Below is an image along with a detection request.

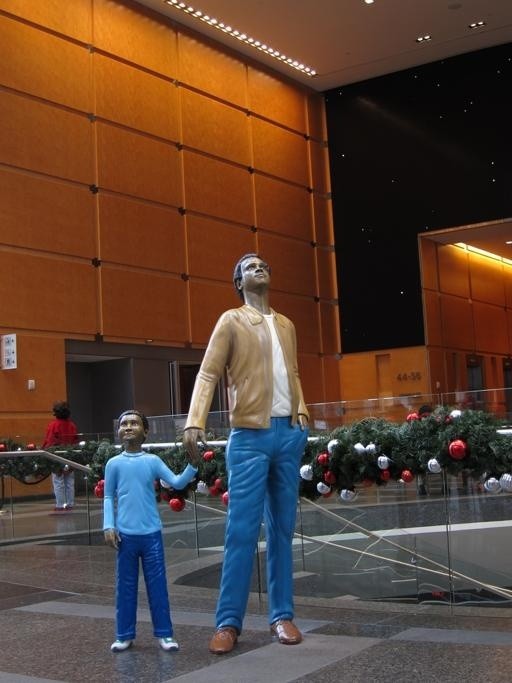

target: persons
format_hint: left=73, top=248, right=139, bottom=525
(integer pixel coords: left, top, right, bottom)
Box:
left=184, top=253, right=310, bottom=653
left=103, top=409, right=200, bottom=652
left=42, top=400, right=78, bottom=509
